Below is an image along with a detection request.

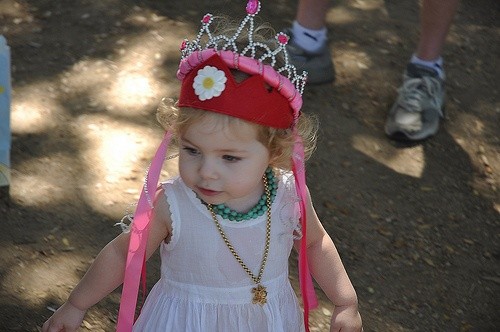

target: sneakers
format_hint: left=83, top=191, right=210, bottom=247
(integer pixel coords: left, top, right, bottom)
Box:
left=273, top=28, right=337, bottom=92
left=384, top=72, right=448, bottom=141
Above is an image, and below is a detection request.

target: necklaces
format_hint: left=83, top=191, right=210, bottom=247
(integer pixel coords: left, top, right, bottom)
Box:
left=192, top=167, right=278, bottom=304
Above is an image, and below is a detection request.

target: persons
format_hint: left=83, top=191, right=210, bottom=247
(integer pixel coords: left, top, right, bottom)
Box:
left=238, top=0, right=459, bottom=142
left=41, top=16, right=362, bottom=332
